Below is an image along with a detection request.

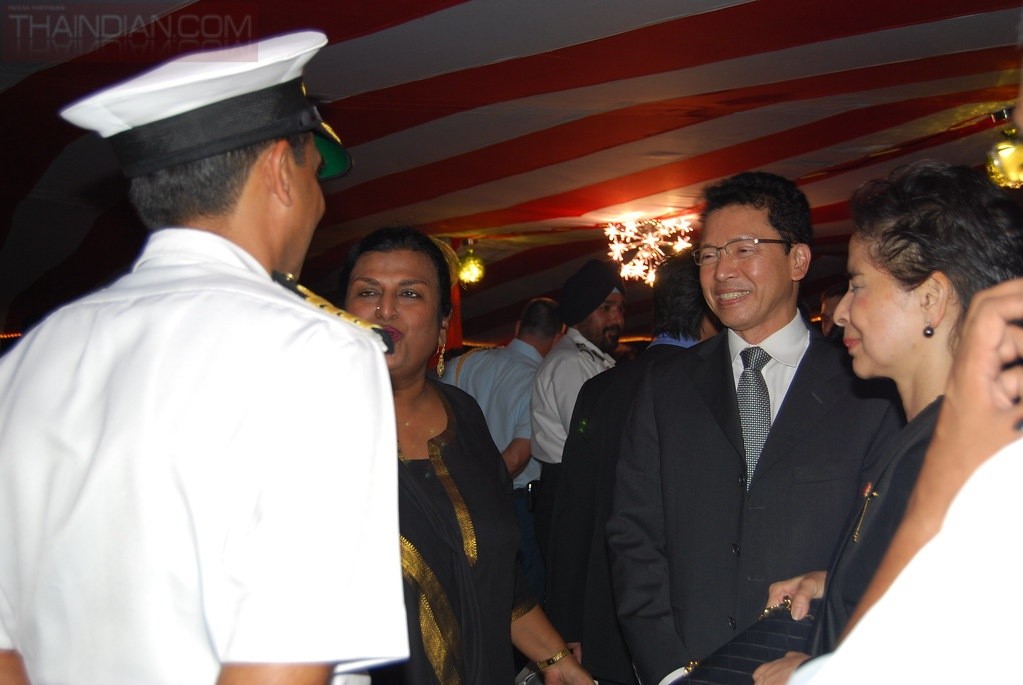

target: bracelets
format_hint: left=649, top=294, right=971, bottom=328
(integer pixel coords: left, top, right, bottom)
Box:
left=537, top=648, right=570, bottom=670
left=659, top=661, right=699, bottom=685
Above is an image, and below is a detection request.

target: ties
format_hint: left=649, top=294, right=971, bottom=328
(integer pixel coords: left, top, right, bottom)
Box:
left=736, top=346, right=773, bottom=493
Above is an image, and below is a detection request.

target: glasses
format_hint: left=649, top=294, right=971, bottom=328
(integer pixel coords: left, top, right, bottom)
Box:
left=693, top=237, right=792, bottom=267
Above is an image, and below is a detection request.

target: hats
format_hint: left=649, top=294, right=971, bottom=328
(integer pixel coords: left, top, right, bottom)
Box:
left=49, top=30, right=357, bottom=185
left=558, top=258, right=617, bottom=326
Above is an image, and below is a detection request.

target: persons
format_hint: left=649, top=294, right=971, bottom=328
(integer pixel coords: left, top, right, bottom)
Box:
left=536, top=251, right=722, bottom=685
left=603, top=172, right=903, bottom=685
left=821, top=286, right=847, bottom=337
left=440, top=296, right=564, bottom=600
left=1, top=32, right=410, bottom=684
left=807, top=157, right=1023, bottom=658
left=336, top=224, right=602, bottom=685
left=525, top=258, right=626, bottom=500
left=787, top=276, right=1023, bottom=685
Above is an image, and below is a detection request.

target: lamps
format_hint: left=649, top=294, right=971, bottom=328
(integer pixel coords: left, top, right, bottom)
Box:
left=602, top=220, right=694, bottom=287
left=987, top=108, right=1023, bottom=189
left=456, top=239, right=484, bottom=285
